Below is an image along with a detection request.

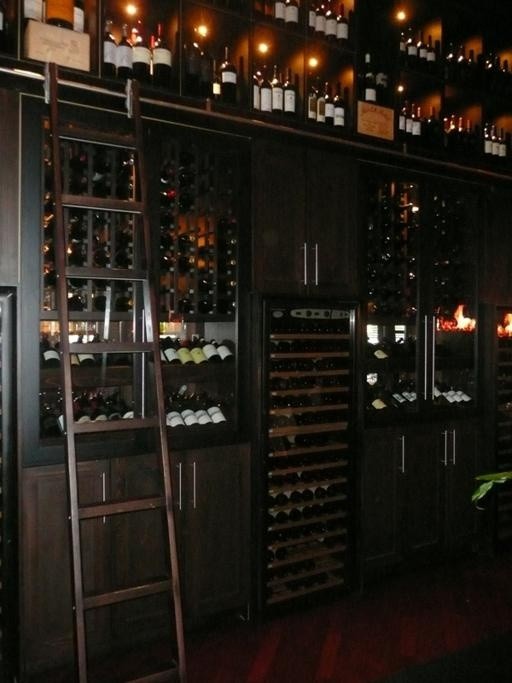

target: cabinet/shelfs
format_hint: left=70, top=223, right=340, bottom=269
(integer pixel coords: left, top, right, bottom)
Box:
left=0, top=0, right=511, bottom=682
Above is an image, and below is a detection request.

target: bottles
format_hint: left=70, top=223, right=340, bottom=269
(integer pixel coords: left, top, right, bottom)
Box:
left=23, top=1, right=88, bottom=33
left=365, top=180, right=473, bottom=412
left=401, top=28, right=437, bottom=66
left=103, top=18, right=174, bottom=90
left=262, top=318, right=353, bottom=593
left=357, top=51, right=392, bottom=105
left=183, top=37, right=238, bottom=103
left=307, top=71, right=347, bottom=126
left=256, top=1, right=349, bottom=42
left=444, top=42, right=509, bottom=159
left=400, top=99, right=438, bottom=146
left=41, top=128, right=238, bottom=313
left=253, top=64, right=298, bottom=114
left=38, top=336, right=234, bottom=434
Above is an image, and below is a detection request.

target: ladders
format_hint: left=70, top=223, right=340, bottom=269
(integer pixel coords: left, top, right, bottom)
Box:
left=46, top=61, right=188, bottom=682
